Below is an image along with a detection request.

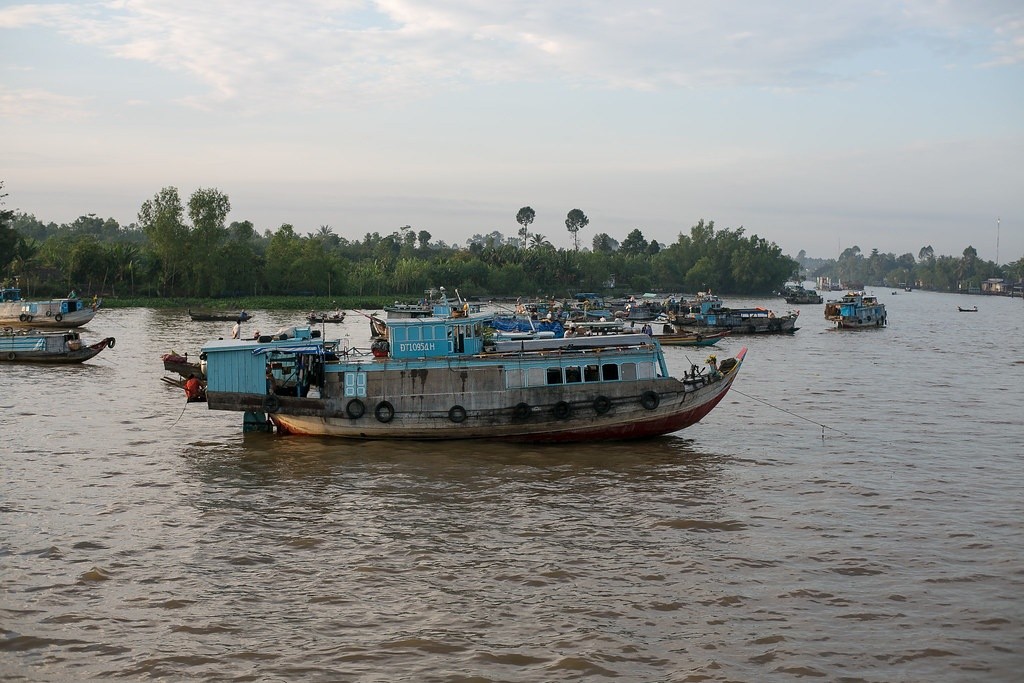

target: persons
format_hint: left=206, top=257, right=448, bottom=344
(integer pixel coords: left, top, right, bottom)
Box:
left=309, top=310, right=317, bottom=319
left=185, top=374, right=204, bottom=399
left=335, top=308, right=340, bottom=317
left=240, top=308, right=247, bottom=316
left=564, top=314, right=652, bottom=337
left=231, top=320, right=241, bottom=339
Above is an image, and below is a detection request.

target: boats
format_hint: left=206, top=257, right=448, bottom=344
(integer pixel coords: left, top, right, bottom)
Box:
left=0, top=275, right=102, bottom=329
left=158, top=311, right=749, bottom=445
left=354, top=282, right=802, bottom=348
left=0, top=324, right=117, bottom=365
left=187, top=308, right=254, bottom=321
left=822, top=289, right=888, bottom=328
left=304, top=311, right=346, bottom=324
left=771, top=281, right=825, bottom=305
left=956, top=305, right=979, bottom=313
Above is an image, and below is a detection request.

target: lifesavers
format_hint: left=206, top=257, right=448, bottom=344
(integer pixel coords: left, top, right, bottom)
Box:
left=108, top=337, right=115, bottom=348
left=594, top=396, right=611, bottom=413
left=55, top=314, right=62, bottom=322
left=449, top=405, right=467, bottom=423
left=20, top=314, right=32, bottom=323
left=641, top=390, right=660, bottom=410
left=263, top=395, right=280, bottom=413
left=727, top=324, right=733, bottom=330
left=858, top=319, right=862, bottom=323
left=697, top=336, right=702, bottom=342
left=750, top=326, right=756, bottom=331
left=46, top=311, right=51, bottom=317
left=375, top=400, right=395, bottom=423
left=867, top=316, right=871, bottom=321
left=7, top=352, right=16, bottom=360
left=554, top=400, right=570, bottom=419
left=346, top=399, right=365, bottom=418
left=515, top=402, right=530, bottom=418
